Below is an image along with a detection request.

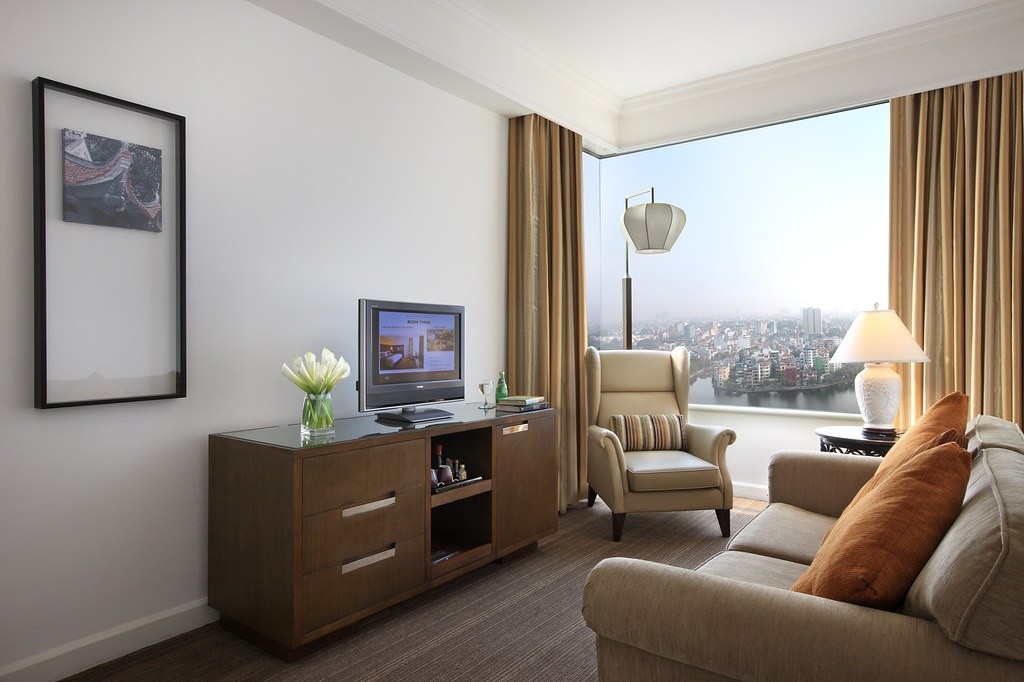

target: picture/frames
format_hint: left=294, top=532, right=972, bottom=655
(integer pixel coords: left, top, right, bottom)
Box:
left=31, top=76, right=188, bottom=408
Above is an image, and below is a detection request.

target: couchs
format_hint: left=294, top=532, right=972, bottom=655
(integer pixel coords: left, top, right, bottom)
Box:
left=582, top=415, right=1024, bottom=682
left=584, top=346, right=735, bottom=542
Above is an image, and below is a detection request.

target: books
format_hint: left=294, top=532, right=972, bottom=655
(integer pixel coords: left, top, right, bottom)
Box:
left=496, top=396, right=549, bottom=412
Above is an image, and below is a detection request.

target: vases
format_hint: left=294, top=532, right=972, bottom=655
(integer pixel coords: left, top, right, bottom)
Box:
left=302, top=393, right=337, bottom=438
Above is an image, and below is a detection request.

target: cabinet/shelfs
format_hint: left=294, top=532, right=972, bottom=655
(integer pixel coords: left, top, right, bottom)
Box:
left=206, top=402, right=561, bottom=661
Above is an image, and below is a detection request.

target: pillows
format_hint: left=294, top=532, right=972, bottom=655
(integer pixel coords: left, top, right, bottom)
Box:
left=610, top=416, right=690, bottom=452
left=792, top=444, right=971, bottom=607
left=873, top=391, right=973, bottom=472
left=822, top=431, right=969, bottom=538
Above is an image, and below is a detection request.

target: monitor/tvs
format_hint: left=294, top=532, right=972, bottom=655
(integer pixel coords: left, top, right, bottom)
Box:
left=357, top=299, right=466, bottom=423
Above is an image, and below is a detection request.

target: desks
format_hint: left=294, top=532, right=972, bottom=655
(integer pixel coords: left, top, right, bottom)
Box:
left=814, top=426, right=900, bottom=457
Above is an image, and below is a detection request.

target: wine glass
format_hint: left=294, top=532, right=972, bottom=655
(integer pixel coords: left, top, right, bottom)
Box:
left=478, top=380, right=494, bottom=409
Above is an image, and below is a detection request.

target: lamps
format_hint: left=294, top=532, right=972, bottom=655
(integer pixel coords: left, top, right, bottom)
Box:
left=827, top=303, right=933, bottom=436
left=622, top=188, right=686, bottom=349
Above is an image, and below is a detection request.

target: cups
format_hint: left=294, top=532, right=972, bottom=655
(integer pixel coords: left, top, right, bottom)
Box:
left=437, top=465, right=453, bottom=484
left=431, top=469, right=438, bottom=486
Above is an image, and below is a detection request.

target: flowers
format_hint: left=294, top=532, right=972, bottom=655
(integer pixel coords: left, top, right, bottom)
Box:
left=279, top=349, right=350, bottom=427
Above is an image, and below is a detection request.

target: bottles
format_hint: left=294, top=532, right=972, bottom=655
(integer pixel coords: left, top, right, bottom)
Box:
left=435, top=444, right=467, bottom=490
left=495, top=371, right=509, bottom=405
